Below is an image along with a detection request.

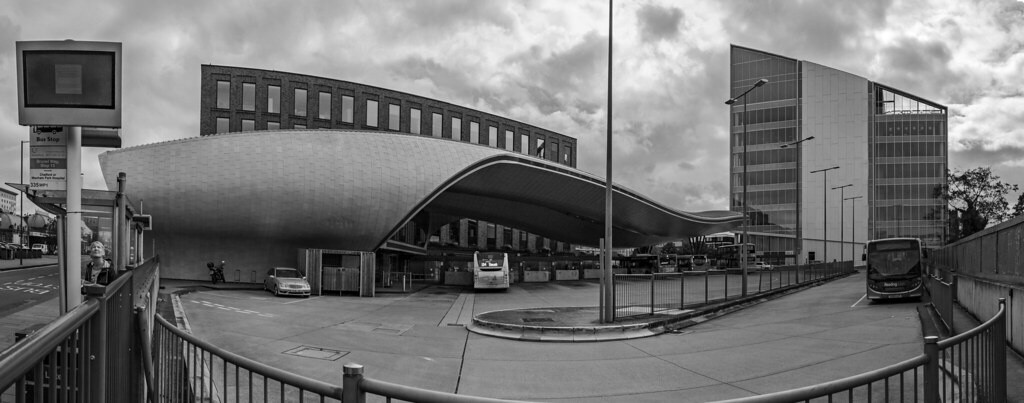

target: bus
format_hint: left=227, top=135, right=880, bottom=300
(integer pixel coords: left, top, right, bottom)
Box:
left=715, top=243, right=757, bottom=269
left=627, top=253, right=678, bottom=280
left=677, top=254, right=708, bottom=273
left=862, top=237, right=928, bottom=302
left=473, top=251, right=510, bottom=292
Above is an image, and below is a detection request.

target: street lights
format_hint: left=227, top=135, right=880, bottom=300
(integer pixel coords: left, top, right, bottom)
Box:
left=831, top=183, right=854, bottom=277
left=779, top=134, right=815, bottom=285
left=843, top=195, right=864, bottom=273
left=810, top=166, right=841, bottom=278
left=724, top=77, right=770, bottom=298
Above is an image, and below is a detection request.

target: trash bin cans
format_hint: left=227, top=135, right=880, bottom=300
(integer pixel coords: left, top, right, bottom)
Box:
left=15, top=322, right=81, bottom=403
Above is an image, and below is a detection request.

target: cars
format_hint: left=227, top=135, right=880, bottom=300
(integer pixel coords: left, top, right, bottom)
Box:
left=264, top=267, right=311, bottom=298
left=0, top=241, right=49, bottom=256
left=756, top=262, right=774, bottom=269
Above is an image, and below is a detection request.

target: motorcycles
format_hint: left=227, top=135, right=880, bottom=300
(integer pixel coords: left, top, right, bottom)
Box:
left=207, top=260, right=227, bottom=284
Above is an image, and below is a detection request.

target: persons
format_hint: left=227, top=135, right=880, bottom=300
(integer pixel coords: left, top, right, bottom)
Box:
left=86, top=240, right=116, bottom=288
